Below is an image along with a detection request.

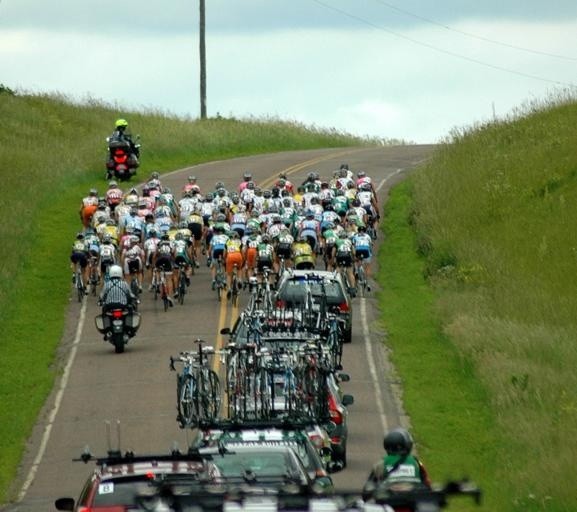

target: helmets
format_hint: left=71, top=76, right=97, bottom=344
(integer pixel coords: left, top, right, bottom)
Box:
left=109, top=264, right=123, bottom=279
left=76, top=171, right=202, bottom=244
left=384, top=428, right=412, bottom=454
left=206, top=164, right=372, bottom=243
left=115, top=119, right=128, bottom=129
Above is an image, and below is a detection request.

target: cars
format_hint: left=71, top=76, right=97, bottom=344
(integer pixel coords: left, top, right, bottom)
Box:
left=55, top=346, right=354, bottom=512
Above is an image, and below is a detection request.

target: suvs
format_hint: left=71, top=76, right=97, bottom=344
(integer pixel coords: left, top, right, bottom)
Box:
left=273, top=265, right=357, bottom=344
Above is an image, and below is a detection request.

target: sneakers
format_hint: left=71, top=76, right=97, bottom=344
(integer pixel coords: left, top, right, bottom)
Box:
left=167, top=296, right=174, bottom=307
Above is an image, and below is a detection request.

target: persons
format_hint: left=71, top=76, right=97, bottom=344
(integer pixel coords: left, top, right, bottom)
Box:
left=69, top=163, right=377, bottom=347
left=105, top=118, right=138, bottom=180
left=362, top=428, right=427, bottom=492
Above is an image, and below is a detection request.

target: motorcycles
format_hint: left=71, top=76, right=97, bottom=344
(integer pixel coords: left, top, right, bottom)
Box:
left=90, top=279, right=142, bottom=354
left=363, top=476, right=433, bottom=511
left=105, top=133, right=142, bottom=181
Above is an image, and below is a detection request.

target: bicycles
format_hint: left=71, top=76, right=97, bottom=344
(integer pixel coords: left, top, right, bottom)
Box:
left=70, top=206, right=372, bottom=312
left=169, top=276, right=344, bottom=430
